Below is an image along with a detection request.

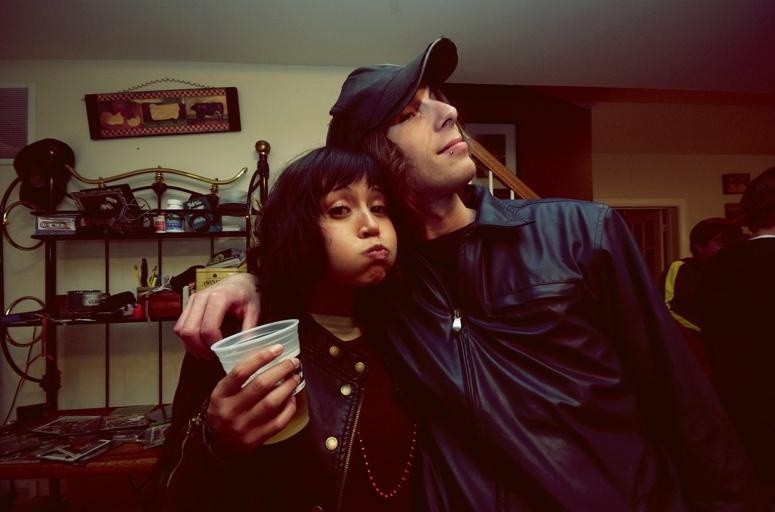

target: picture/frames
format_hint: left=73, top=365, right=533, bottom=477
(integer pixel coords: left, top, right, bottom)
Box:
left=461, top=122, right=517, bottom=191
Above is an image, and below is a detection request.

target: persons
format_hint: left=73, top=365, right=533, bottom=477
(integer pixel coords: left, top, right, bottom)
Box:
left=142, top=143, right=467, bottom=511
left=699, top=160, right=774, bottom=486
left=658, top=213, right=744, bottom=334
left=173, top=32, right=775, bottom=511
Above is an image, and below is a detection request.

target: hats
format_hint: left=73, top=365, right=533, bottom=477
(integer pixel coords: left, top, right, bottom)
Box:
left=326, top=36, right=460, bottom=150
left=13, top=138, right=77, bottom=211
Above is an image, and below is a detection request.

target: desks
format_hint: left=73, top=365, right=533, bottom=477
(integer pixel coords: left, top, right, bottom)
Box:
left=0, top=415, right=172, bottom=512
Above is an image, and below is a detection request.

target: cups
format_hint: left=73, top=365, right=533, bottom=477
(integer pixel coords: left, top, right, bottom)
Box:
left=210, top=317, right=310, bottom=445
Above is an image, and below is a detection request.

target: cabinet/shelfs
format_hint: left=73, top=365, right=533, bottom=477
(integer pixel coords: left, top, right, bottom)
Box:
left=0, top=137, right=272, bottom=413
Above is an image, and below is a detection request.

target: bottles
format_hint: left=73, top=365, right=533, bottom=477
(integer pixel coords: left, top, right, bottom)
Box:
left=156, top=199, right=185, bottom=233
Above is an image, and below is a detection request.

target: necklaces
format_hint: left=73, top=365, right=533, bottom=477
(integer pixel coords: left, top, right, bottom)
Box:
left=353, top=419, right=420, bottom=501
